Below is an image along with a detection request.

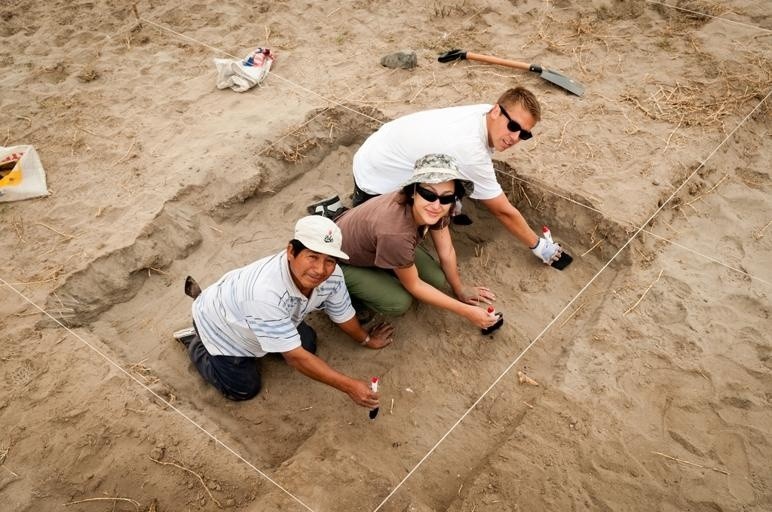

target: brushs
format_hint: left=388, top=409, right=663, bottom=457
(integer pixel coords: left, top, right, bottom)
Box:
left=481, top=307, right=504, bottom=335
left=185, top=276, right=202, bottom=300
left=543, top=226, right=573, bottom=271
left=369, top=377, right=379, bottom=419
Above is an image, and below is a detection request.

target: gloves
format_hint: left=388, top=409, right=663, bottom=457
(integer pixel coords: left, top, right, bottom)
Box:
left=450, top=196, right=463, bottom=217
left=529, top=238, right=559, bottom=264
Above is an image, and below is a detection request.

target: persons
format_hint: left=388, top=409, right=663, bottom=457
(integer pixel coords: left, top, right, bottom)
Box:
left=306, top=85, right=563, bottom=266
left=329, top=153, right=501, bottom=331
left=172, top=213, right=394, bottom=411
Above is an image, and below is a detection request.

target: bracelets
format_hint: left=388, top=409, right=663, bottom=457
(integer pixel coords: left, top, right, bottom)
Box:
left=359, top=333, right=371, bottom=347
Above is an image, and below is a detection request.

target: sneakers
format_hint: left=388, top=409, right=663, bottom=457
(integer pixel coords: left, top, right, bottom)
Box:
left=174, top=327, right=196, bottom=350
left=307, top=194, right=343, bottom=217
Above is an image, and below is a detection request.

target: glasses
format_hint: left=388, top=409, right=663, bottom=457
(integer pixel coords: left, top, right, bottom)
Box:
left=499, top=104, right=533, bottom=141
left=416, top=185, right=456, bottom=205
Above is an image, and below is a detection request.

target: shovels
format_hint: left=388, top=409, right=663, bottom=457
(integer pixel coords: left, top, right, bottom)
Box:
left=437, top=50, right=587, bottom=97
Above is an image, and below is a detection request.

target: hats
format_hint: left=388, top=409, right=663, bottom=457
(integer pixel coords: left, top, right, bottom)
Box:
left=293, top=214, right=351, bottom=260
left=401, top=152, right=475, bottom=202
